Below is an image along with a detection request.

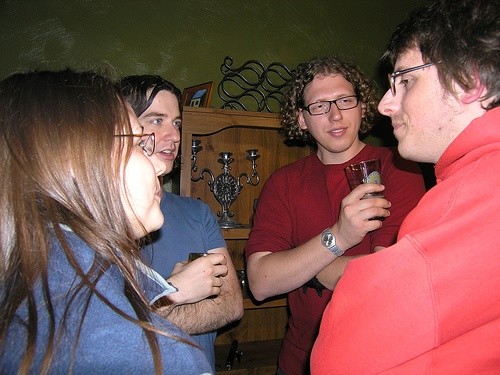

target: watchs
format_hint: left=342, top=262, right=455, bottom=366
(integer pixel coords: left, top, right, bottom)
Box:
left=320, top=226, right=345, bottom=258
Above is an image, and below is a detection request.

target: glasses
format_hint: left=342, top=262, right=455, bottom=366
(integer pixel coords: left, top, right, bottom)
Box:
left=303, top=95, right=360, bottom=115
left=114, top=132, right=155, bottom=156
left=387, top=60, right=441, bottom=97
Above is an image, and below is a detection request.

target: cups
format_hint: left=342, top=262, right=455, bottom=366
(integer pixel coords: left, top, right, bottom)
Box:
left=188, top=252, right=219, bottom=298
left=344, top=157, right=386, bottom=221
left=236, top=270, right=251, bottom=298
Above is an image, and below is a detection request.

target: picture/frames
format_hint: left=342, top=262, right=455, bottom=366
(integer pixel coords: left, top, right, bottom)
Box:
left=179, top=80, right=215, bottom=108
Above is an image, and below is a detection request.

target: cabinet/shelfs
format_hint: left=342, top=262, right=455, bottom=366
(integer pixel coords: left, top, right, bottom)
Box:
left=172, top=105, right=317, bottom=375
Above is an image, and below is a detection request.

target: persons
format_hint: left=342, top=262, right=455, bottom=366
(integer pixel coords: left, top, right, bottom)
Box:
left=310, top=0, right=500, bottom=375
left=244, top=55, right=427, bottom=375
left=0, top=65, right=243, bottom=375
